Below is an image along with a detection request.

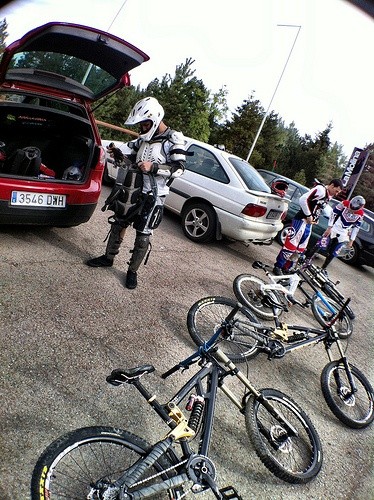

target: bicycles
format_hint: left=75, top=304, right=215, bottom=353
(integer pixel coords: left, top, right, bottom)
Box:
left=283, top=242, right=355, bottom=319
left=30, top=302, right=324, bottom=500
left=186, top=295, right=374, bottom=429
left=232, top=244, right=354, bottom=338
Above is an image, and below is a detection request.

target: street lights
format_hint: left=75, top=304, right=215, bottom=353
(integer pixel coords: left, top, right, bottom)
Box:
left=246, top=25, right=302, bottom=162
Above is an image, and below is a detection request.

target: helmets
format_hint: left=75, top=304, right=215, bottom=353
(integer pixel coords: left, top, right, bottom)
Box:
left=350, top=195, right=364, bottom=214
left=121, top=98, right=164, bottom=140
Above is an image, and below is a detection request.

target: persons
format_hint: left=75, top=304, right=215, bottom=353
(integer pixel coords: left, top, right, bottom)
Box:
left=274, top=179, right=344, bottom=277
left=305, top=195, right=365, bottom=276
left=87, top=97, right=187, bottom=289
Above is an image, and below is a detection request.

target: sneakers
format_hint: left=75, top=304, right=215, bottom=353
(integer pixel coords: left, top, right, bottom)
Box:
left=126, top=269, right=136, bottom=292
left=86, top=254, right=118, bottom=269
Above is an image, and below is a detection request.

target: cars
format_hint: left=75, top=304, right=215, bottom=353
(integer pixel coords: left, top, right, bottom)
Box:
left=102, top=133, right=292, bottom=244
left=257, top=168, right=334, bottom=253
left=328, top=196, right=374, bottom=267
left=0, top=21, right=151, bottom=228
